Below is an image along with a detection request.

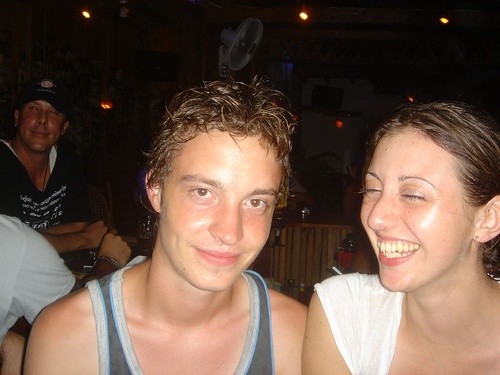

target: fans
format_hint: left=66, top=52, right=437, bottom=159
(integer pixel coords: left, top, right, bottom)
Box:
left=219, top=17, right=264, bottom=80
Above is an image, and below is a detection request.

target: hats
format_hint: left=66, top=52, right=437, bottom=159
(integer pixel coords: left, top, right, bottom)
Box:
left=17, top=72, right=71, bottom=112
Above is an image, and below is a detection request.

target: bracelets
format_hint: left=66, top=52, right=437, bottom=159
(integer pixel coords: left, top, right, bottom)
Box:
left=93, top=256, right=121, bottom=271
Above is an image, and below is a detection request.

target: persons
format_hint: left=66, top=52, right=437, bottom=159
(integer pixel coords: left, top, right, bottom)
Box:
left=23, top=73, right=309, bottom=375
left=300, top=100, right=500, bottom=375
left=0, top=213, right=132, bottom=375
left=0, top=70, right=110, bottom=253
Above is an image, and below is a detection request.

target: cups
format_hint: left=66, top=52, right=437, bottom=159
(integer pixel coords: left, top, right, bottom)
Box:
left=138, top=212, right=156, bottom=239
left=299, top=277, right=306, bottom=294
